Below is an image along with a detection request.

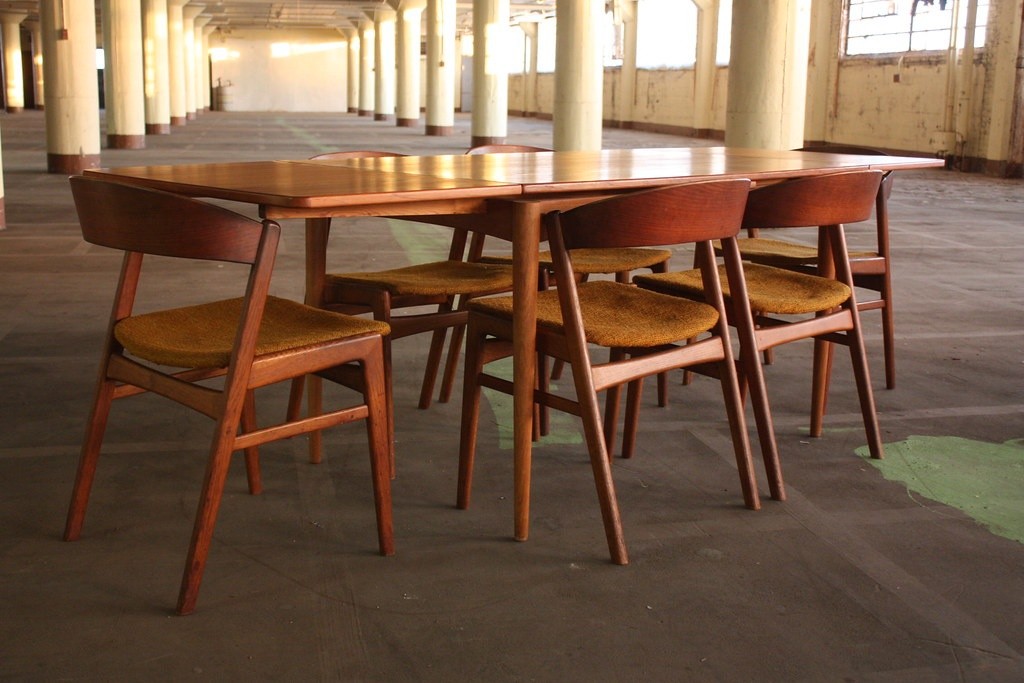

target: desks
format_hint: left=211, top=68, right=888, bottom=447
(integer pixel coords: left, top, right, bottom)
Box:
left=81, top=148, right=944, bottom=497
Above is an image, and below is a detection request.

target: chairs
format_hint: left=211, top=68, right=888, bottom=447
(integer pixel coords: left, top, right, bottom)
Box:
left=61, top=145, right=893, bottom=612
